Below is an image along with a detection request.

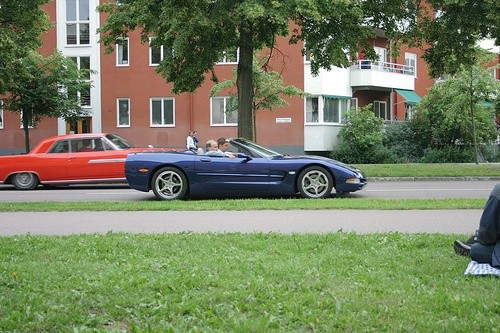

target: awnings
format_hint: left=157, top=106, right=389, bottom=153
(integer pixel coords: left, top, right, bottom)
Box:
left=394, top=90, right=422, bottom=104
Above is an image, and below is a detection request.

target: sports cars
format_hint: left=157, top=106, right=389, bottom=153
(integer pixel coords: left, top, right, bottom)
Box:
left=124, top=137, right=368, bottom=201
left=0, top=132, right=189, bottom=191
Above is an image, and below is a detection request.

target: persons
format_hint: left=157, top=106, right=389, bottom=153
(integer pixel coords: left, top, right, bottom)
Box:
left=187, top=130, right=196, bottom=149
left=78, top=139, right=94, bottom=151
left=204, top=140, right=235, bottom=159
left=94, top=139, right=104, bottom=150
left=216, top=137, right=239, bottom=156
left=454, top=183, right=500, bottom=268
left=192, top=131, right=199, bottom=144
